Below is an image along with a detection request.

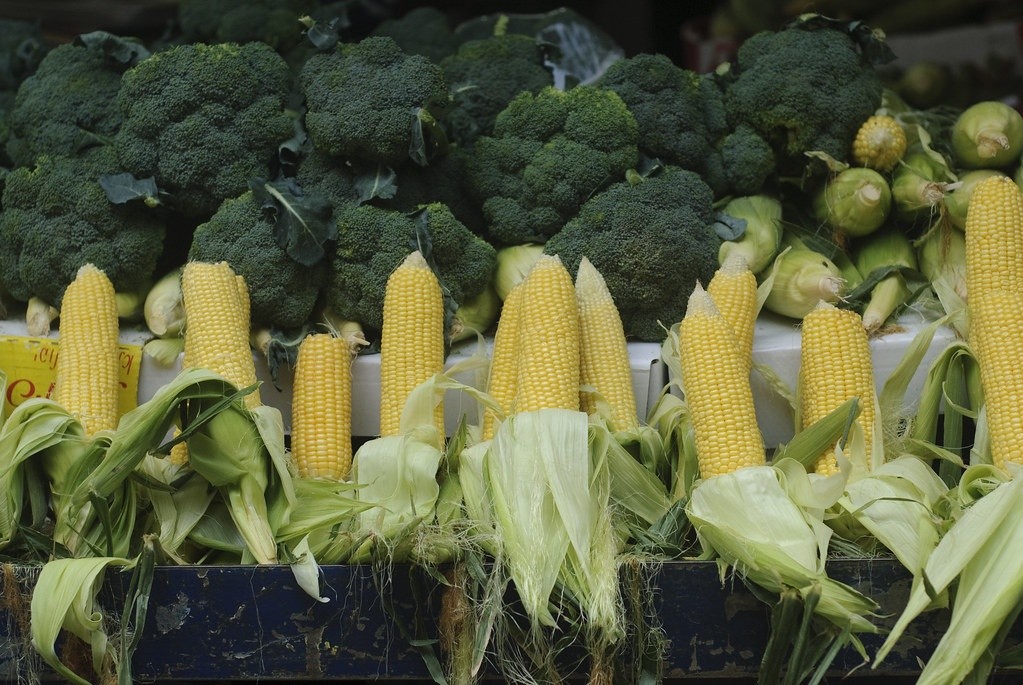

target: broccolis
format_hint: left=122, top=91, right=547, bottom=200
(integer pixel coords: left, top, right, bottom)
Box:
left=0, top=0, right=907, bottom=344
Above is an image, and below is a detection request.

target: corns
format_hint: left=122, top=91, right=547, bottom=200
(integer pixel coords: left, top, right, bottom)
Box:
left=52, top=261, right=1022, bottom=543
left=715, top=86, right=1023, bottom=334
